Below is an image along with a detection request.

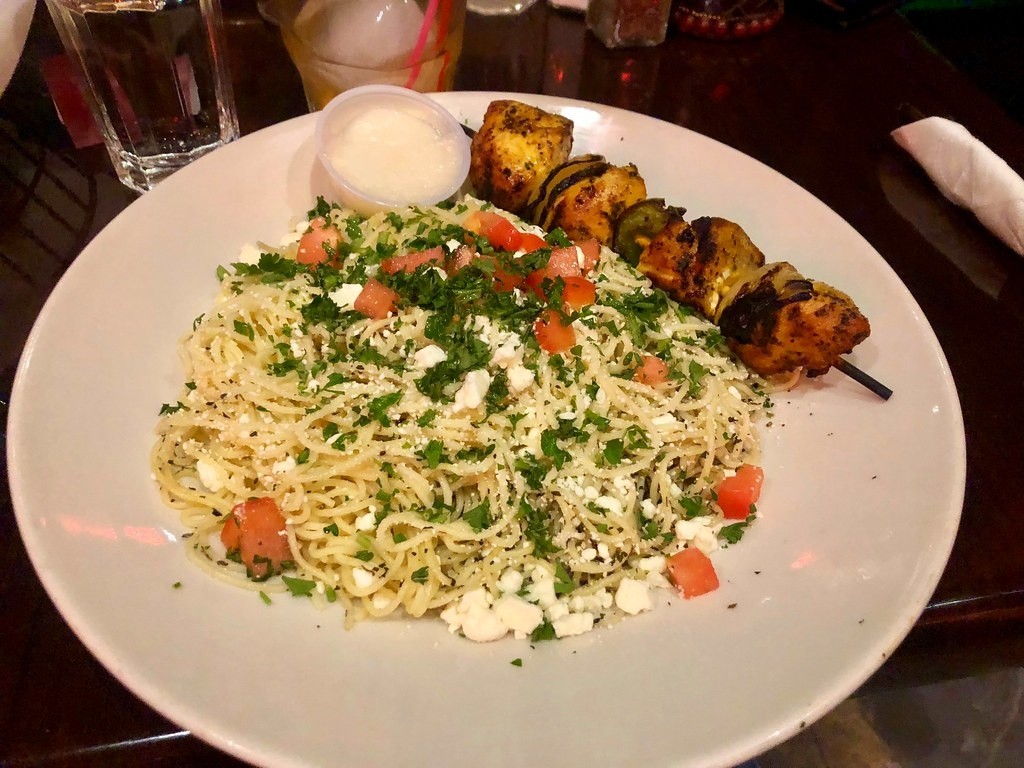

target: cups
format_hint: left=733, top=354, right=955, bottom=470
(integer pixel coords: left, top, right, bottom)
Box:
left=260, top=0, right=468, bottom=114
left=44, top=0, right=241, bottom=196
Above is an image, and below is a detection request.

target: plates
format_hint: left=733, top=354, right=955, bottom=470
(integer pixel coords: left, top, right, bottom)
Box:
left=4, top=90, right=969, bottom=768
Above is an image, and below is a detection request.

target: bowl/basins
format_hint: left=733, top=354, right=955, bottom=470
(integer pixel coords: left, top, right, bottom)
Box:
left=315, top=84, right=472, bottom=220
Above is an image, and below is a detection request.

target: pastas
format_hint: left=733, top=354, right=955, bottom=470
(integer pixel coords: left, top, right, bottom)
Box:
left=148, top=186, right=800, bottom=633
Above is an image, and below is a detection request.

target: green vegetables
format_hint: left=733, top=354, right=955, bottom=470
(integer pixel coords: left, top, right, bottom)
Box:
left=160, top=192, right=774, bottom=670
